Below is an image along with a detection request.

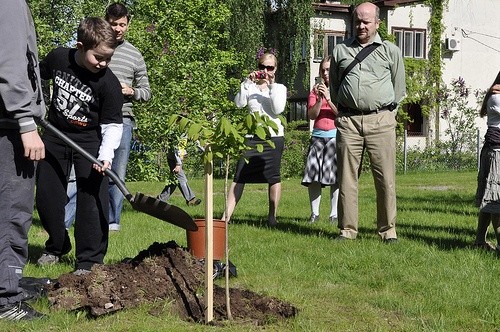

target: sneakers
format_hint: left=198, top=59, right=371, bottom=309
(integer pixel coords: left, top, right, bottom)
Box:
left=1, top=302, right=49, bottom=323
left=37, top=246, right=72, bottom=266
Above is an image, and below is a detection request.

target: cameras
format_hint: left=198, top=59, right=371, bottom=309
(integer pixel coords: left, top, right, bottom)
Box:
left=256, top=72, right=267, bottom=79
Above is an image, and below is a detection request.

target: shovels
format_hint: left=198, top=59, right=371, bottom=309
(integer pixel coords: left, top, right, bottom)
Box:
left=40, top=119, right=199, bottom=231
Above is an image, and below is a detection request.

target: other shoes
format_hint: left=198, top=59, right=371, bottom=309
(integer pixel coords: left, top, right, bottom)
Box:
left=186, top=198, right=201, bottom=207
left=109, top=224, right=121, bottom=233
left=474, top=242, right=497, bottom=251
left=309, top=215, right=320, bottom=223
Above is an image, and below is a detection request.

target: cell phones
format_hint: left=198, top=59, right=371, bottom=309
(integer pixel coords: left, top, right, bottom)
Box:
left=315, top=77, right=324, bottom=85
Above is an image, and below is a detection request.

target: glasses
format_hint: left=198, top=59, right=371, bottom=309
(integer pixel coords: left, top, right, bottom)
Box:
left=259, top=63, right=277, bottom=71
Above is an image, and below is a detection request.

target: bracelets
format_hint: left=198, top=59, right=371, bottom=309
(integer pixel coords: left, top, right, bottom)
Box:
left=326, top=97, right=331, bottom=102
left=317, top=98, right=322, bottom=103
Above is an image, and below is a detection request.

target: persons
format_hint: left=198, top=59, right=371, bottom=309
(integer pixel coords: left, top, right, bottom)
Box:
left=328, top=2, right=406, bottom=244
left=154, top=138, right=201, bottom=206
left=302, top=56, right=345, bottom=225
left=38, top=3, right=151, bottom=238
left=30, top=17, right=123, bottom=276
left=220, top=54, right=288, bottom=228
left=1, top=1, right=50, bottom=325
left=473, top=71, right=500, bottom=259
